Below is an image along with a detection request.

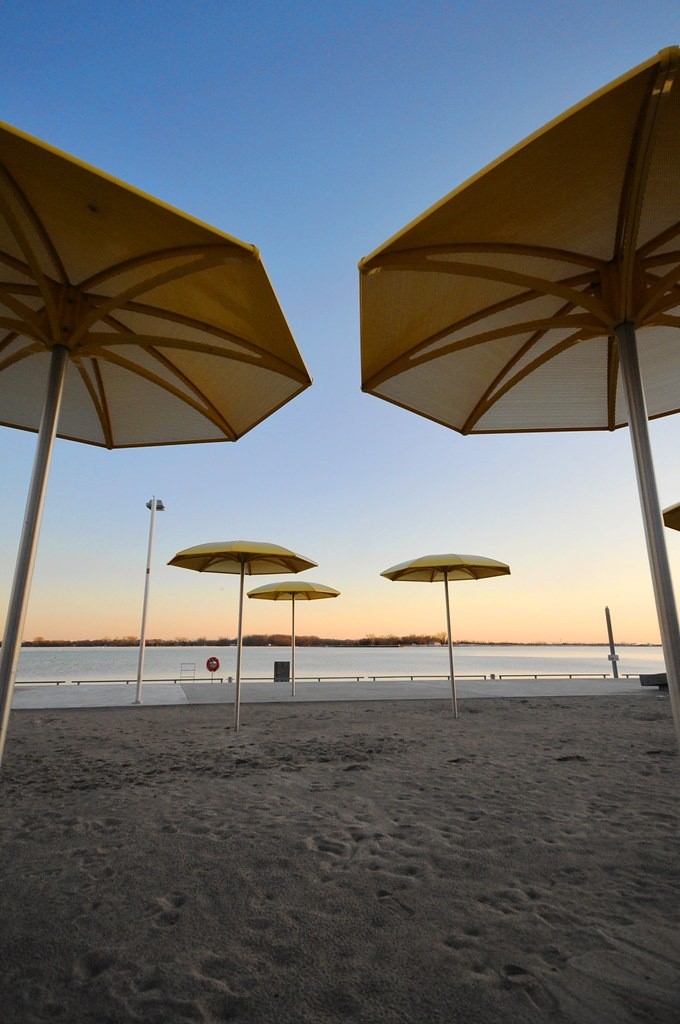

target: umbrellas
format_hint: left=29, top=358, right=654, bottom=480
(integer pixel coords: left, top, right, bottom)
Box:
left=357, top=45, right=680, bottom=723
left=380, top=553, right=511, bottom=719
left=0, top=117, right=312, bottom=756
left=167, top=541, right=318, bottom=732
left=662, top=503, right=680, bottom=532
left=247, top=581, right=341, bottom=695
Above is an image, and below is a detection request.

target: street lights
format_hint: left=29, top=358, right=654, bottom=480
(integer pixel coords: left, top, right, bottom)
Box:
left=132, top=497, right=166, bottom=704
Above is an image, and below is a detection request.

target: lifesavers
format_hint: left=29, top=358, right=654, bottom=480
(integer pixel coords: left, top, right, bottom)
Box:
left=206, top=657, right=220, bottom=672
